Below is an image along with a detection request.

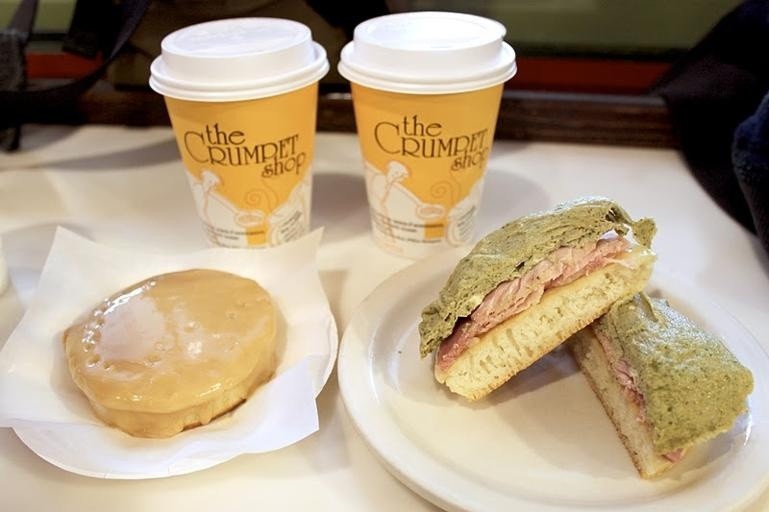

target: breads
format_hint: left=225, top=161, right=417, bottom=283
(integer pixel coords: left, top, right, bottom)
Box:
left=567, top=293, right=754, bottom=480
left=62, top=269, right=274, bottom=438
left=420, top=199, right=654, bottom=401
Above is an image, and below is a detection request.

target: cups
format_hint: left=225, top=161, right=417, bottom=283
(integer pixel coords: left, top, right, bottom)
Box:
left=148, top=17, right=332, bottom=251
left=336, top=10, right=516, bottom=262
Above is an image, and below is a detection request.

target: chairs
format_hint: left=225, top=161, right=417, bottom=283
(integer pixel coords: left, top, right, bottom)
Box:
left=0, top=0, right=150, bottom=149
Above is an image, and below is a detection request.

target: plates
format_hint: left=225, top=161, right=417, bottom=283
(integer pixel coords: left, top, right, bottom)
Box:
left=0, top=266, right=338, bottom=482
left=339, top=245, right=769, bottom=511
